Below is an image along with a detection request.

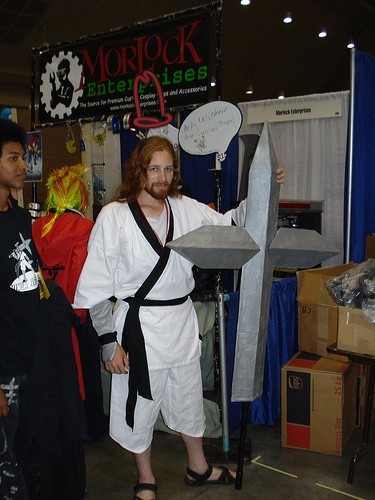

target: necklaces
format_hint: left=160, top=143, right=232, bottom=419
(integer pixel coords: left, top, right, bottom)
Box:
left=142, top=200, right=169, bottom=251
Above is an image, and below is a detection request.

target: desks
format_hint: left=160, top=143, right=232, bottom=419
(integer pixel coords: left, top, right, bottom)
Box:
left=326, top=342, right=375, bottom=484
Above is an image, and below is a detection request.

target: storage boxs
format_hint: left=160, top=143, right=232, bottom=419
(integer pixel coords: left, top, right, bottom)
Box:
left=296, top=262, right=360, bottom=364
left=337, top=305, right=375, bottom=359
left=281, top=351, right=357, bottom=456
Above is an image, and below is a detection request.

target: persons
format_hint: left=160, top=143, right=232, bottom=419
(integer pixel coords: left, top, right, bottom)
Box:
left=0, top=117, right=40, bottom=500
left=72, top=136, right=286, bottom=500
left=31, top=163, right=96, bottom=323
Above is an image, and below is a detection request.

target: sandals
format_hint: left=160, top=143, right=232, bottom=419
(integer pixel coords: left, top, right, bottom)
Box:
left=133, top=480, right=157, bottom=500
left=185, top=464, right=235, bottom=485
